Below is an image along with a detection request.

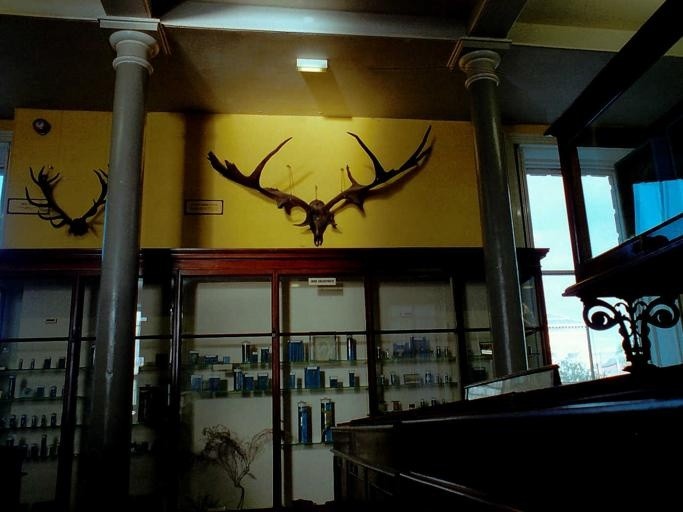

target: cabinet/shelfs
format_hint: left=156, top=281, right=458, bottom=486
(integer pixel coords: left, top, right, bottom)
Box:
left=320, top=396, right=334, bottom=443
left=298, top=402, right=309, bottom=445
left=186, top=332, right=455, bottom=411
left=2, top=355, right=65, bottom=456
left=0, top=246, right=550, bottom=512
